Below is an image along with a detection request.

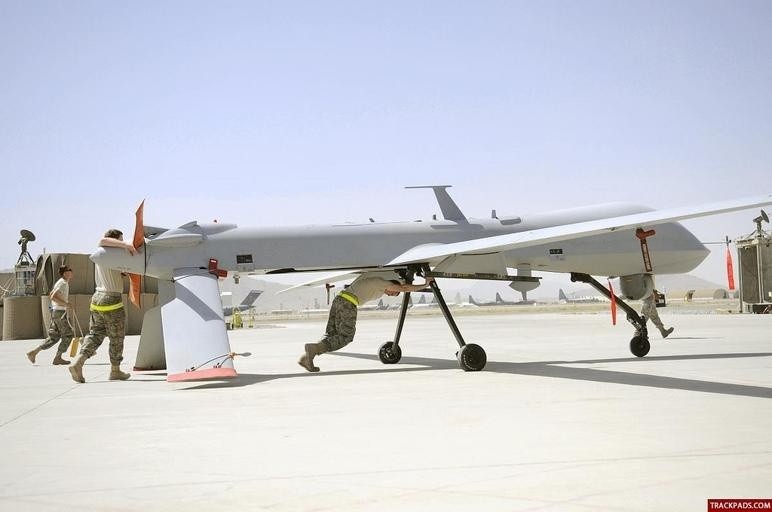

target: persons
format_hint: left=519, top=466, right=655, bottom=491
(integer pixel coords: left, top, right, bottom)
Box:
left=299, top=277, right=434, bottom=372
left=68, top=229, right=136, bottom=383
left=632, top=292, right=674, bottom=340
left=26, top=265, right=74, bottom=365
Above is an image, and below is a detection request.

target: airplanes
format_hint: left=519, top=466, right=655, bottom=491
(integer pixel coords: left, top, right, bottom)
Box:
left=359, top=286, right=612, bottom=312
left=221, top=287, right=264, bottom=317
left=88, top=180, right=772, bottom=387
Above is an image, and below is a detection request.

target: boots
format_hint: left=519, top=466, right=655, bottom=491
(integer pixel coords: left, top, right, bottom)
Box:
left=26, top=347, right=43, bottom=364
left=53, top=352, right=70, bottom=365
left=68, top=352, right=88, bottom=383
left=109, top=364, right=130, bottom=380
left=299, top=342, right=328, bottom=372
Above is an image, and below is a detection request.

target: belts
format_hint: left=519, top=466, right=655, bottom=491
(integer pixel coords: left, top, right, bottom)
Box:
left=90, top=301, right=124, bottom=311
left=337, top=292, right=359, bottom=306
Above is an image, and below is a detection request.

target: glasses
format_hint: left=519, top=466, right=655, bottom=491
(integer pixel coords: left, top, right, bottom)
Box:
left=66, top=269, right=72, bottom=271
